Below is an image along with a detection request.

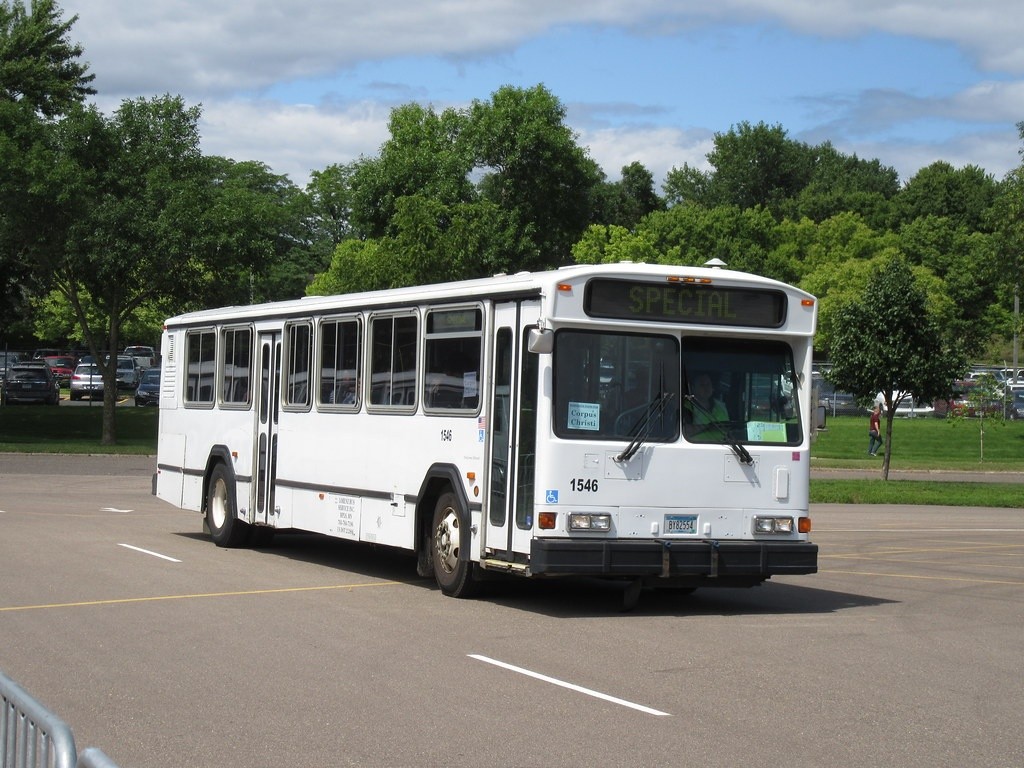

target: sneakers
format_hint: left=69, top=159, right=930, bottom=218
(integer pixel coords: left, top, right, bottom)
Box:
left=869, top=452, right=877, bottom=457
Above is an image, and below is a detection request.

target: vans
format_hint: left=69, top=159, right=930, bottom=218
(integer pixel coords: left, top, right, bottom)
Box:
left=124, top=346, right=156, bottom=375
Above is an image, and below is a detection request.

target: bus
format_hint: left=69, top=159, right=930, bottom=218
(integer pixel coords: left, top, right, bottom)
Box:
left=153, top=254, right=823, bottom=598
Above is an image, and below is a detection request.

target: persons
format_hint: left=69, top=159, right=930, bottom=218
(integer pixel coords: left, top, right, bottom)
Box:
left=675, top=373, right=736, bottom=442
left=868, top=409, right=882, bottom=457
left=329, top=367, right=355, bottom=404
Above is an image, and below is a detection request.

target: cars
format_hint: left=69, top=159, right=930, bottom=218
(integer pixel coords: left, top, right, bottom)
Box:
left=812, top=359, right=1024, bottom=423
left=44, top=355, right=78, bottom=389
left=69, top=363, right=119, bottom=401
left=138, top=367, right=161, bottom=387
left=32, top=349, right=53, bottom=366
left=133, top=374, right=159, bottom=407
left=79, top=356, right=100, bottom=365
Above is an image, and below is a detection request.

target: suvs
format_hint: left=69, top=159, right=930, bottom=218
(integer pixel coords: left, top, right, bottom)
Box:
left=3, top=362, right=61, bottom=406
left=104, top=354, right=141, bottom=390
left=0, top=352, right=30, bottom=390
